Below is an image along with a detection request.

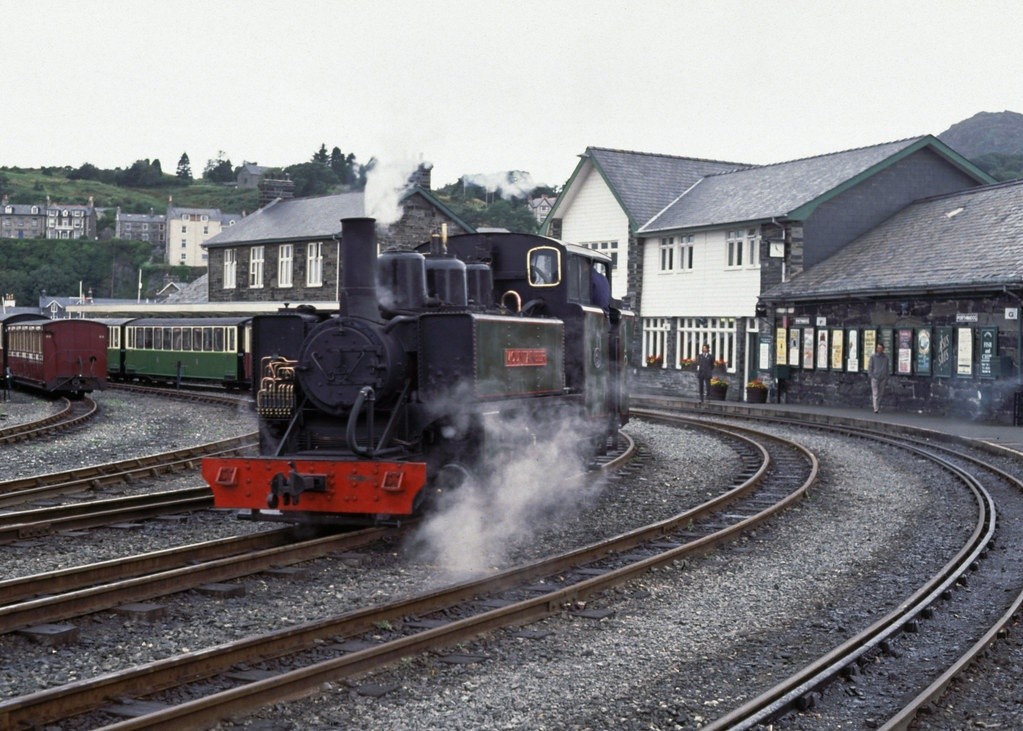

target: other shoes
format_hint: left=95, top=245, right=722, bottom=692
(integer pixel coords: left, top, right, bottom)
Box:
left=873, top=408, right=881, bottom=414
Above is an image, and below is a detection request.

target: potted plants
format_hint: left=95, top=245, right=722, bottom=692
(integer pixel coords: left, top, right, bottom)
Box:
left=709, top=376, right=731, bottom=400
left=713, top=359, right=727, bottom=374
left=646, top=355, right=662, bottom=368
left=746, top=381, right=768, bottom=404
left=680, top=359, right=698, bottom=371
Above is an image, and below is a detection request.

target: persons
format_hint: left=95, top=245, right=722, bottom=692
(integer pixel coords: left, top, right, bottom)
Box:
left=867, top=343, right=889, bottom=414
left=697, top=344, right=715, bottom=403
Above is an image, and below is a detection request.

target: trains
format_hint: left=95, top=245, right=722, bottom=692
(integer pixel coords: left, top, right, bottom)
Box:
left=104, top=214, right=632, bottom=530
left=0, top=310, right=111, bottom=403
